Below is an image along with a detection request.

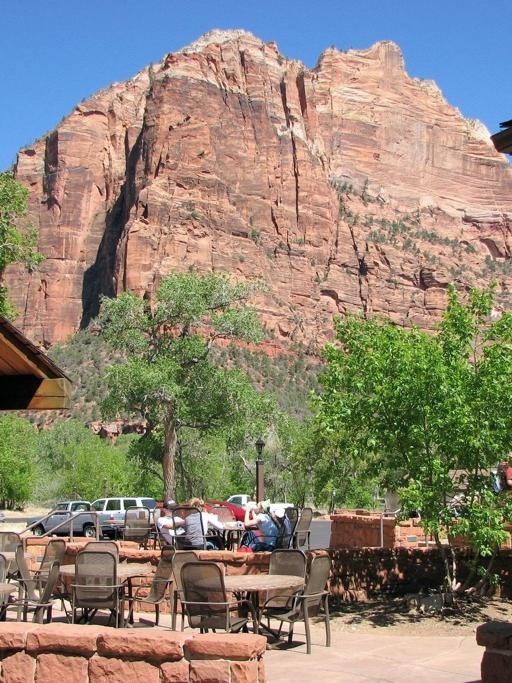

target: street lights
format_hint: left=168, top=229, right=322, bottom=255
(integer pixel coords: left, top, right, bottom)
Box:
left=255, top=437, right=265, bottom=503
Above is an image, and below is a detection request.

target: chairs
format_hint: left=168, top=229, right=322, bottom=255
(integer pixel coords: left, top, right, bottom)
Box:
left=178, top=562, right=258, bottom=634
left=6, top=539, right=65, bottom=612
left=91, top=510, right=120, bottom=541
left=1, top=531, right=26, bottom=621
left=172, top=508, right=224, bottom=550
left=69, top=550, right=123, bottom=627
left=173, top=551, right=217, bottom=633
left=260, top=556, right=330, bottom=655
left=250, top=549, right=306, bottom=637
left=289, top=508, right=315, bottom=549
left=121, top=507, right=150, bottom=551
left=83, top=541, right=115, bottom=624
left=149, top=504, right=175, bottom=550
left=209, top=507, right=241, bottom=551
left=16, top=544, right=77, bottom=623
left=246, top=507, right=298, bottom=552
left=107, top=546, right=175, bottom=628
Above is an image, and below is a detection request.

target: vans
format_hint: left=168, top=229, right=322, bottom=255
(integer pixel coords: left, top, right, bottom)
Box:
left=92, top=497, right=155, bottom=524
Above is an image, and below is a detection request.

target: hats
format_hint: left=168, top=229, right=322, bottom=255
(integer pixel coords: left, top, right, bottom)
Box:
left=261, top=501, right=271, bottom=513
left=163, top=498, right=178, bottom=508
left=273, top=508, right=285, bottom=518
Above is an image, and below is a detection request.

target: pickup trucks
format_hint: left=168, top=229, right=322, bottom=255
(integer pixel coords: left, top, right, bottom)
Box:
left=227, top=494, right=294, bottom=530
left=27, top=500, right=145, bottom=537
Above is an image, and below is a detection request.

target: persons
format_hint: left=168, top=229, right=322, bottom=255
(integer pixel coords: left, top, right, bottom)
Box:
left=270, top=503, right=294, bottom=548
left=242, top=500, right=281, bottom=553
left=183, top=497, right=226, bottom=550
left=496, top=450, right=511, bottom=489
left=156, top=497, right=214, bottom=550
